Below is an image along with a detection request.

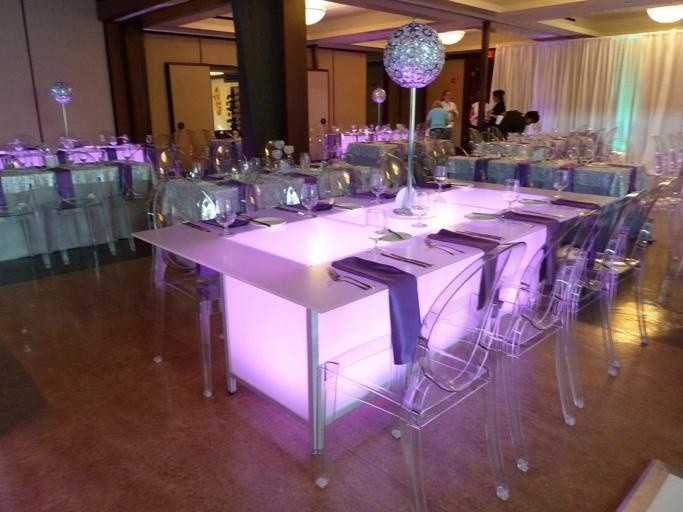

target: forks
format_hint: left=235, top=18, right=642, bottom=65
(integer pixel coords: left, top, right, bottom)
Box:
left=327, top=267, right=372, bottom=290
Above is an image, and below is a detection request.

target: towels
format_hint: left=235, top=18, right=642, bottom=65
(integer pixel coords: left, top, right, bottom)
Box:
left=348, top=192, right=396, bottom=199
left=197, top=218, right=249, bottom=276
left=100, top=147, right=117, bottom=162
left=288, top=202, right=332, bottom=213
left=551, top=198, right=601, bottom=270
left=110, top=162, right=132, bottom=194
left=0, top=176, right=7, bottom=206
left=427, top=228, right=500, bottom=311
left=217, top=179, right=258, bottom=213
left=499, top=210, right=559, bottom=285
left=417, top=182, right=452, bottom=189
left=46, top=167, right=75, bottom=209
left=57, top=150, right=66, bottom=165
left=284, top=172, right=317, bottom=186
left=331, top=256, right=422, bottom=365
left=334, top=166, right=362, bottom=194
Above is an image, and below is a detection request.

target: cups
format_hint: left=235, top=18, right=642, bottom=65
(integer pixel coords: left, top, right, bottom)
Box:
left=298, top=152, right=312, bottom=170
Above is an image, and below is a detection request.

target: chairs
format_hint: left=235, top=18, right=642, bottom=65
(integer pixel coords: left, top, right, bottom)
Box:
left=59, top=133, right=81, bottom=146
left=518, top=187, right=647, bottom=408
left=260, top=138, right=293, bottom=168
left=156, top=146, right=194, bottom=181
left=149, top=178, right=223, bottom=398
left=436, top=124, right=646, bottom=196
left=370, top=152, right=415, bottom=188
left=317, top=162, right=361, bottom=198
left=559, top=178, right=673, bottom=367
left=239, top=164, right=300, bottom=213
left=315, top=241, right=528, bottom=512
left=109, top=147, right=154, bottom=252
left=212, top=145, right=248, bottom=173
left=52, top=151, right=116, bottom=266
left=652, top=129, right=683, bottom=207
left=424, top=207, right=602, bottom=473
left=0, top=156, right=52, bottom=270
left=97, top=130, right=114, bottom=145
left=16, top=134, right=36, bottom=144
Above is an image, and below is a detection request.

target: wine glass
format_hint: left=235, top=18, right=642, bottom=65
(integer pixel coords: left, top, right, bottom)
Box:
left=351, top=123, right=393, bottom=133
left=367, top=174, right=388, bottom=204
left=241, top=156, right=262, bottom=177
left=408, top=192, right=429, bottom=228
left=80, top=134, right=153, bottom=169
left=191, top=161, right=205, bottom=180
left=551, top=169, right=571, bottom=200
left=299, top=183, right=317, bottom=218
left=503, top=178, right=519, bottom=214
left=363, top=209, right=387, bottom=257
left=215, top=197, right=237, bottom=237
left=475, top=126, right=598, bottom=166
left=431, top=166, right=448, bottom=193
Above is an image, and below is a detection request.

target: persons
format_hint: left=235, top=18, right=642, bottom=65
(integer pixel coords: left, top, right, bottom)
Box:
left=425, top=90, right=540, bottom=143
left=174, top=121, right=195, bottom=170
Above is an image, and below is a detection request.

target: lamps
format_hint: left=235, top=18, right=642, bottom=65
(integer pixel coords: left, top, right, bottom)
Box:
left=306, top=4, right=327, bottom=25
left=647, top=4, right=683, bottom=23
left=438, top=31, right=466, bottom=45
left=48, top=80, right=74, bottom=137
left=384, top=17, right=446, bottom=216
left=372, top=87, right=387, bottom=127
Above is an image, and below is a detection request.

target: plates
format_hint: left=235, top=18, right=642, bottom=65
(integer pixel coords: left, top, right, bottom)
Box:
left=519, top=199, right=547, bottom=204
left=465, top=214, right=503, bottom=220
left=334, top=202, right=362, bottom=213
left=251, top=215, right=286, bottom=228
left=370, top=230, right=411, bottom=241
left=450, top=183, right=474, bottom=189
left=250, top=198, right=547, bottom=243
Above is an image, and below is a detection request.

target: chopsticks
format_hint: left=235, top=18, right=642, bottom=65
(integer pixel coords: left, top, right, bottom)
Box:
left=380, top=252, right=433, bottom=268
